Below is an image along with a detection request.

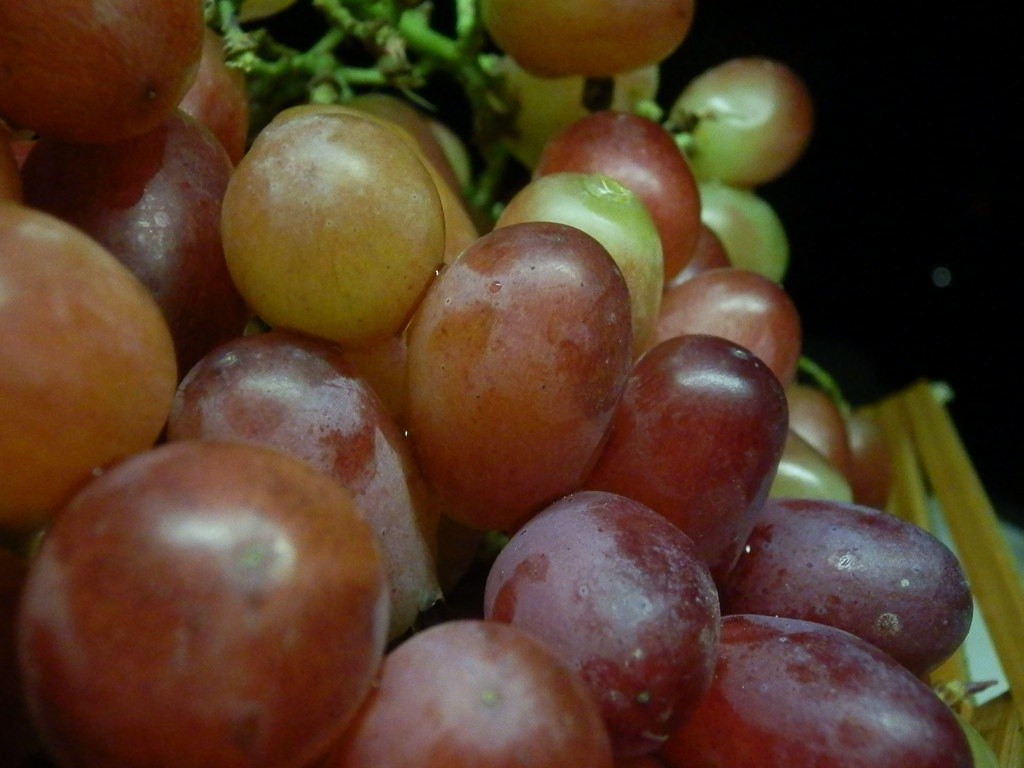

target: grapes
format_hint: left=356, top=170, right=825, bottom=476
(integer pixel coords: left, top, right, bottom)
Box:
left=0, top=0, right=979, bottom=768
